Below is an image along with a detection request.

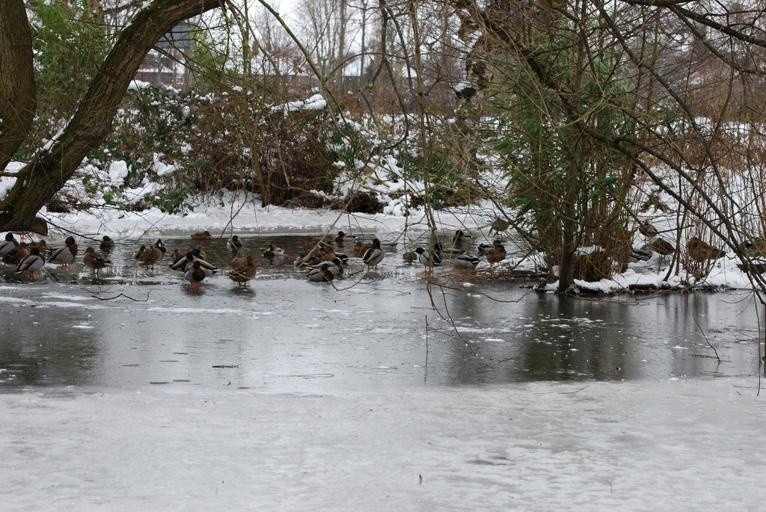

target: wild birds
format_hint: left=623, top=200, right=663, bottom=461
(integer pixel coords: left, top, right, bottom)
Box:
left=224, top=254, right=257, bottom=287
left=0, top=232, right=116, bottom=280
left=431, top=243, right=443, bottom=264
left=652, top=237, right=677, bottom=258
left=305, top=238, right=385, bottom=281
left=475, top=238, right=507, bottom=268
left=227, top=234, right=243, bottom=253
left=262, top=244, right=286, bottom=257
left=411, top=247, right=435, bottom=272
left=452, top=230, right=466, bottom=241
left=685, top=236, right=726, bottom=266
left=191, top=230, right=211, bottom=241
left=639, top=220, right=660, bottom=241
left=135, top=238, right=218, bottom=288
